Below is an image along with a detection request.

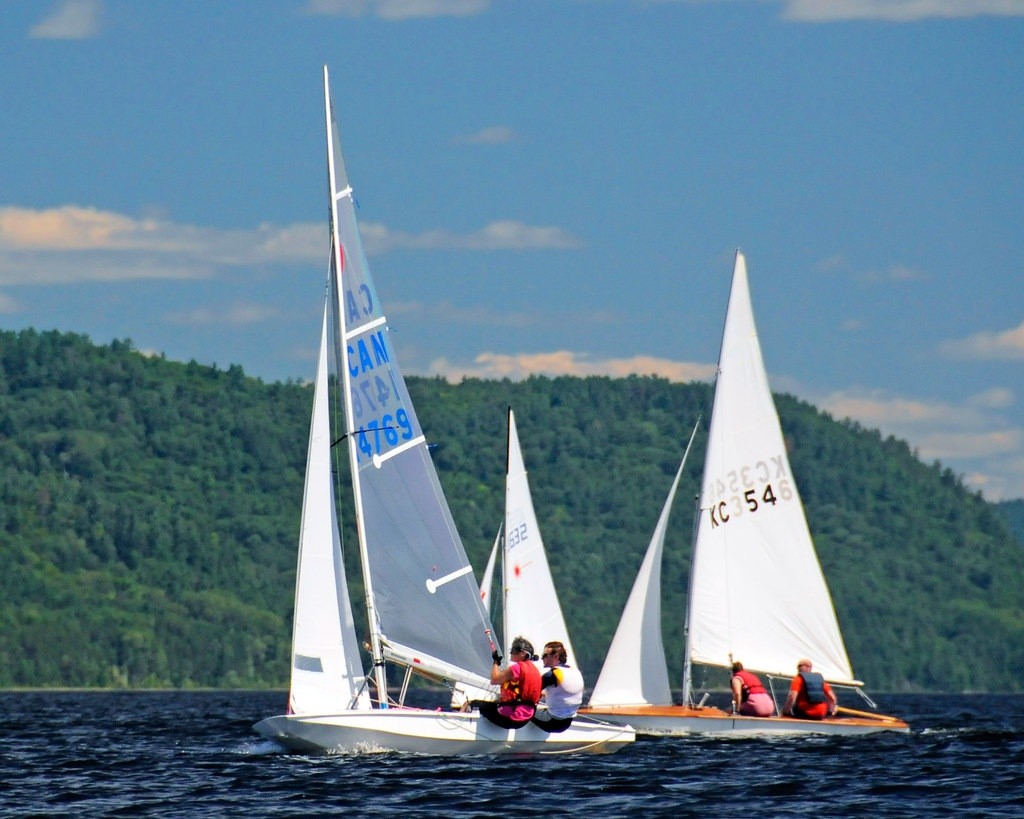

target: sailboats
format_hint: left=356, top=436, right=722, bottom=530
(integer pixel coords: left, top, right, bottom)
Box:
left=250, top=61, right=639, bottom=758
left=579, top=246, right=912, bottom=744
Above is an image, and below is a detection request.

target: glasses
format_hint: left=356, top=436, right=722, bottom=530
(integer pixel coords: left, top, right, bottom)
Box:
left=510, top=647, right=522, bottom=654
left=541, top=653, right=551, bottom=658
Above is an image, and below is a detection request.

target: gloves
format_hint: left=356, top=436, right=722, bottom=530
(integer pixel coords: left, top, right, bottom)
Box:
left=492, top=649, right=503, bottom=665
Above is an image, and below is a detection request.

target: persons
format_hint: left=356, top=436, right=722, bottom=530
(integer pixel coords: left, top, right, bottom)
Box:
left=531, top=641, right=584, bottom=734
left=780, top=661, right=838, bottom=720
left=458, top=637, right=543, bottom=729
left=730, top=662, right=774, bottom=717
